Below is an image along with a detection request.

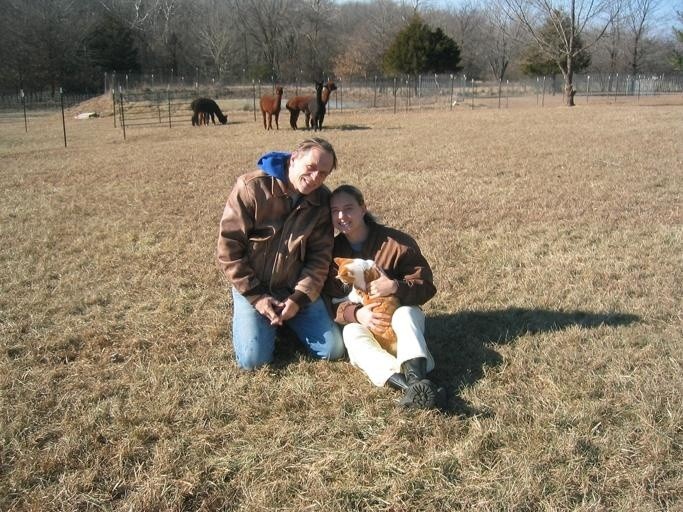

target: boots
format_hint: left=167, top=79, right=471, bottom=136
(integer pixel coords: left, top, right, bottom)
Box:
left=389, top=357, right=447, bottom=415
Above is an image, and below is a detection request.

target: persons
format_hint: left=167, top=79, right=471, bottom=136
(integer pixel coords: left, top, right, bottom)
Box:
left=319, top=184, right=448, bottom=413
left=214, top=136, right=345, bottom=372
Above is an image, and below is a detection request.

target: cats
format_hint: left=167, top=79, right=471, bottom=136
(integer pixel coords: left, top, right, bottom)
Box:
left=333, top=256, right=400, bottom=358
